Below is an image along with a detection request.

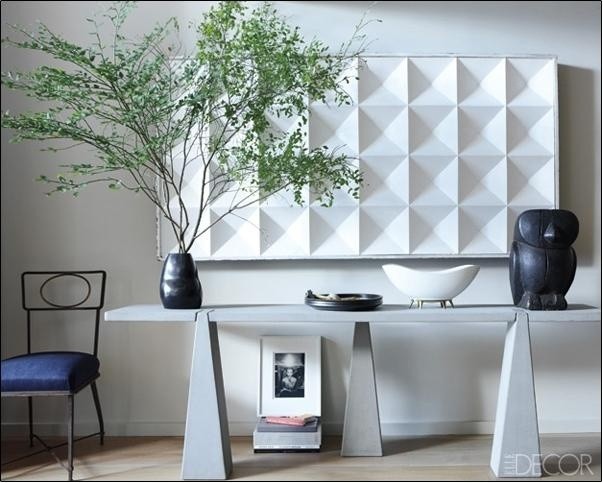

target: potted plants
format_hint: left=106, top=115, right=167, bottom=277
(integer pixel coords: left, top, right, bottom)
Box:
left=0, top=1, right=384, bottom=310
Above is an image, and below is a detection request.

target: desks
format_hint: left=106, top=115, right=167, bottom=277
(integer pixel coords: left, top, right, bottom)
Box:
left=101, top=304, right=601, bottom=480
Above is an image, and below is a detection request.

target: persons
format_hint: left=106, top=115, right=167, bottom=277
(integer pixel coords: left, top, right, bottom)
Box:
left=282, top=368, right=297, bottom=390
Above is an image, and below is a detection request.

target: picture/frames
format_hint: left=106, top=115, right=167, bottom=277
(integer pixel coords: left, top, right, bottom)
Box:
left=258, top=334, right=322, bottom=418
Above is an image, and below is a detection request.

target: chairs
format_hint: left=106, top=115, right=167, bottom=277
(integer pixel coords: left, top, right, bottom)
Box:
left=1, top=269, right=107, bottom=481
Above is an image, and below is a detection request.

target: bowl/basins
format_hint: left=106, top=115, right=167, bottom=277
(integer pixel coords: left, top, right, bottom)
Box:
left=380, top=262, right=479, bottom=301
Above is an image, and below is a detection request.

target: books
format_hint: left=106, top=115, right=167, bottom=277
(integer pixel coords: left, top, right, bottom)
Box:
left=253, top=416, right=322, bottom=453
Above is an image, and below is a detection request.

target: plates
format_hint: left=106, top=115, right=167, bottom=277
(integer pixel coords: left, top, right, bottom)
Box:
left=306, top=291, right=384, bottom=311
